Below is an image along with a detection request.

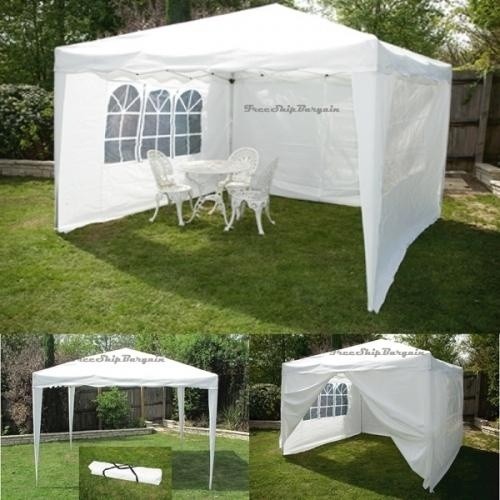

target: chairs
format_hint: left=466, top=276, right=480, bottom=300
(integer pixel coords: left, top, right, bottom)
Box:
left=145, top=145, right=279, bottom=237
